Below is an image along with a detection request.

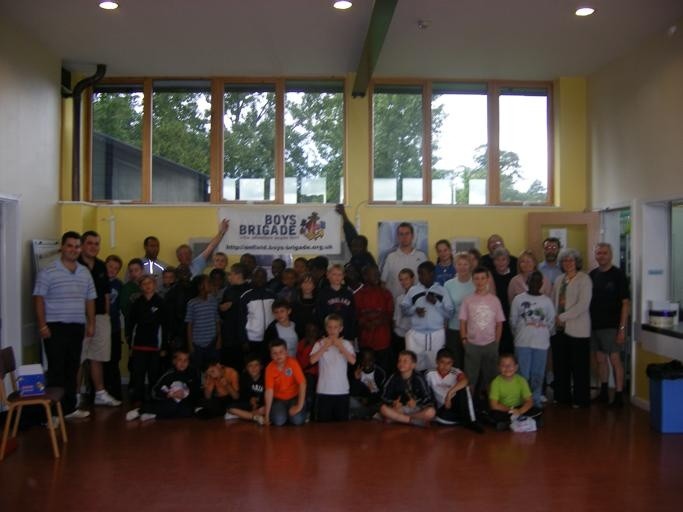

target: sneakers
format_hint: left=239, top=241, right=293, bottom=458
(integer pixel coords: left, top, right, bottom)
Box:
left=408, top=417, right=431, bottom=429
left=47, top=392, right=155, bottom=429
left=225, top=413, right=239, bottom=420
left=253, top=415, right=263, bottom=425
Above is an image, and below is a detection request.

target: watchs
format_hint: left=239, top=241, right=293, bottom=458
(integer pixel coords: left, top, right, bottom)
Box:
left=617, top=325, right=628, bottom=330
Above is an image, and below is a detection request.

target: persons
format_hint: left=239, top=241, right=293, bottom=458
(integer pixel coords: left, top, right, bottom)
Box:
left=352, top=263, right=394, bottom=367
left=125, top=275, right=172, bottom=421
left=308, top=313, right=356, bottom=421
left=224, top=353, right=270, bottom=424
left=587, top=243, right=629, bottom=406
left=423, top=348, right=485, bottom=433
left=182, top=274, right=223, bottom=392
left=74, top=231, right=121, bottom=410
left=194, top=355, right=239, bottom=415
left=233, top=267, right=277, bottom=384
left=508, top=269, right=556, bottom=411
left=483, top=354, right=545, bottom=432
left=263, top=338, right=310, bottom=426
left=31, top=231, right=90, bottom=430
left=551, top=248, right=593, bottom=409
left=348, top=345, right=388, bottom=421
left=103, top=204, right=630, bottom=415
left=151, top=348, right=205, bottom=416
left=398, top=261, right=455, bottom=371
left=379, top=350, right=436, bottom=428
left=315, top=264, right=357, bottom=342
left=457, top=266, right=506, bottom=415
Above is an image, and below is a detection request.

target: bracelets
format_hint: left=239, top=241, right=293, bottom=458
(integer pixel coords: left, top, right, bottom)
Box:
left=460, top=336, right=467, bottom=341
left=37, top=324, right=49, bottom=336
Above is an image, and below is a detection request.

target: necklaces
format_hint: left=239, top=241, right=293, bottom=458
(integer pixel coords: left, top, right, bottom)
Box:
left=562, top=271, right=578, bottom=280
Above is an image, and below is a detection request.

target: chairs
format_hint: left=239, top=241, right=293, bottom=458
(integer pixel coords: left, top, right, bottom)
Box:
left=0, top=346, right=68, bottom=459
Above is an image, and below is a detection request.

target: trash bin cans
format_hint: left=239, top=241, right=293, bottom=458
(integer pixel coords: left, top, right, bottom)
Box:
left=646, top=360, right=683, bottom=432
